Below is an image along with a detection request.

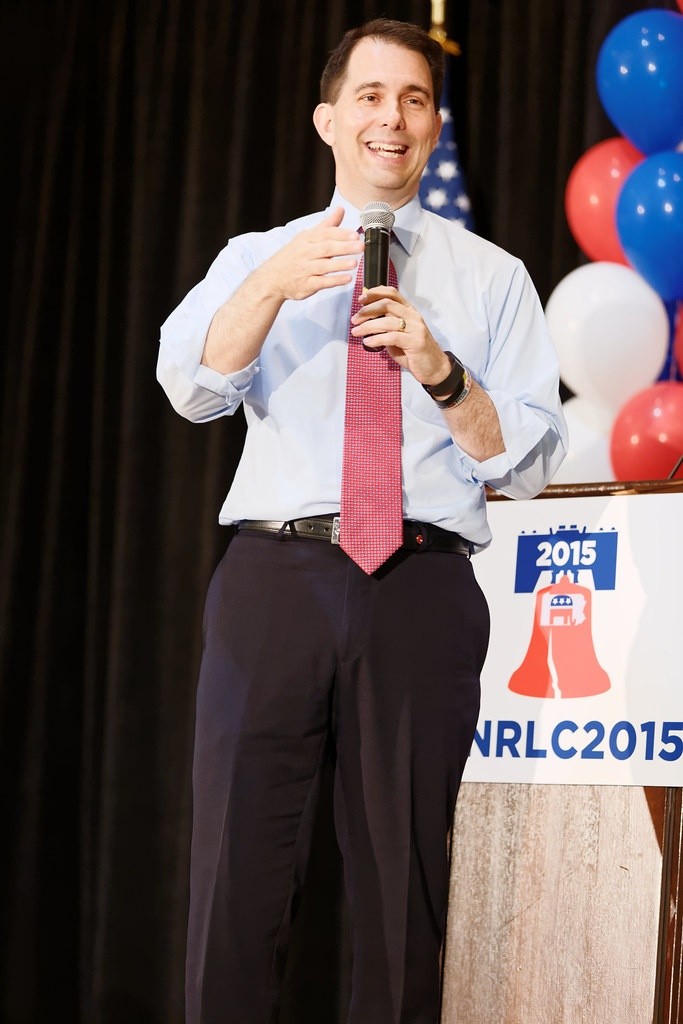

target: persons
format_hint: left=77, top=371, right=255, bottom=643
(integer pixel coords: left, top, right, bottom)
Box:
left=154, top=16, right=568, bottom=1024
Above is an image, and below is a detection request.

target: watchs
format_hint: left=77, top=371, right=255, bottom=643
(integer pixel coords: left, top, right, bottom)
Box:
left=423, top=351, right=465, bottom=395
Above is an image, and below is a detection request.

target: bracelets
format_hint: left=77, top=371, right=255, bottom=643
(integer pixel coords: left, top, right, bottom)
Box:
left=432, top=368, right=473, bottom=409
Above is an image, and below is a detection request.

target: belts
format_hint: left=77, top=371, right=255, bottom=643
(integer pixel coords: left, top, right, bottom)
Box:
left=238, top=512, right=470, bottom=555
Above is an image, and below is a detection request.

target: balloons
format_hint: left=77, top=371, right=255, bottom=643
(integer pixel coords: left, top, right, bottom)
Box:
left=542, top=1, right=682, bottom=486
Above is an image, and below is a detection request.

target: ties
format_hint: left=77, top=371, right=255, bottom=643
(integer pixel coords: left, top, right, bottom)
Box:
left=340, top=225, right=404, bottom=576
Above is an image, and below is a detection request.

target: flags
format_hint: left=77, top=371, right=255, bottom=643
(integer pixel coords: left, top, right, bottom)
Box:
left=418, top=19, right=469, bottom=237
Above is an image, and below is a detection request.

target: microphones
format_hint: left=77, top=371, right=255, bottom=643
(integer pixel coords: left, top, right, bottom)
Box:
left=358, top=200, right=396, bottom=352
